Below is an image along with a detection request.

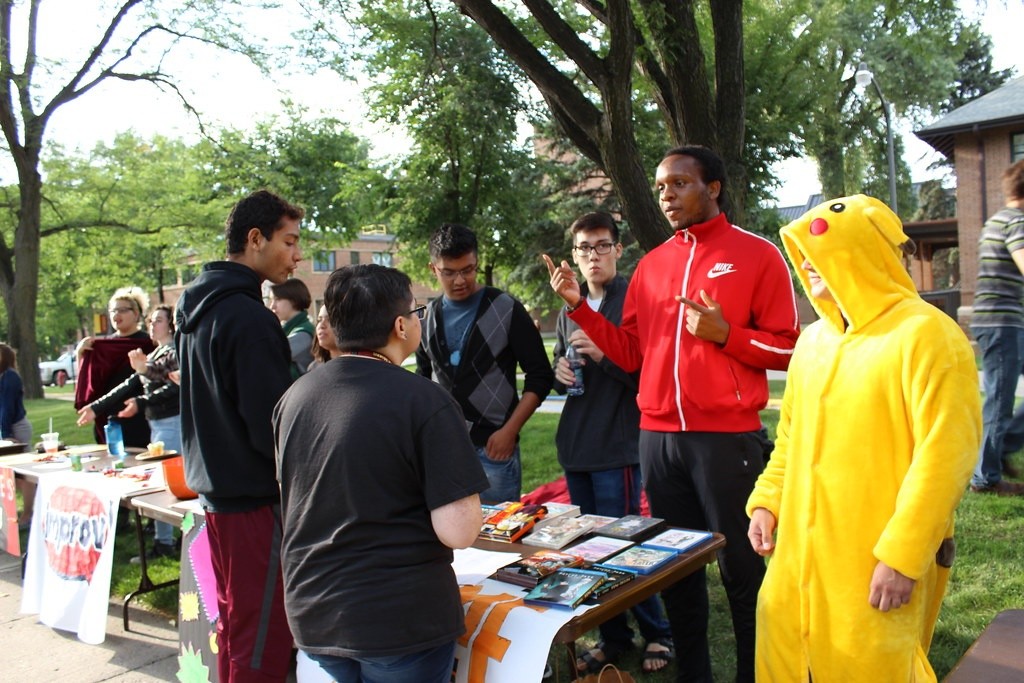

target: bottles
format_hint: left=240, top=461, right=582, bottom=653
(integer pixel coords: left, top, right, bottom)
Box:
left=565, top=341, right=585, bottom=396
left=104, top=414, right=125, bottom=455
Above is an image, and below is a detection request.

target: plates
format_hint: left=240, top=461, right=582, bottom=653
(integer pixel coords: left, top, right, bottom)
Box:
left=32, top=454, right=67, bottom=462
left=135, top=448, right=178, bottom=460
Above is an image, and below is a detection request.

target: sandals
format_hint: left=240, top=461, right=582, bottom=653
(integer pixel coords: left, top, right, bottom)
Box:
left=575, top=640, right=627, bottom=675
left=640, top=636, right=675, bottom=675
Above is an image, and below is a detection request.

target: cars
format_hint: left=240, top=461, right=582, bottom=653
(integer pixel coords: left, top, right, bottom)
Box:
left=39, top=351, right=79, bottom=385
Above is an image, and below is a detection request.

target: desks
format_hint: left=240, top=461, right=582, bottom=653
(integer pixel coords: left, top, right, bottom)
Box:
left=0, top=437, right=726, bottom=683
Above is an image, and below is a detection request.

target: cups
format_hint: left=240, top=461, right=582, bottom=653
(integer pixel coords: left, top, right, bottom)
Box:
left=41, top=433, right=59, bottom=454
left=71, top=455, right=81, bottom=471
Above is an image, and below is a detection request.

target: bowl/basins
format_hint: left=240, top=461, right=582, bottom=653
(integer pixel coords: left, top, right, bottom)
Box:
left=163, top=456, right=199, bottom=500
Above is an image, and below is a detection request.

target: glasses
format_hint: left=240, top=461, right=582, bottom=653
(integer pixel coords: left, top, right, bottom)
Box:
left=107, top=307, right=136, bottom=316
left=433, top=261, right=478, bottom=280
left=396, top=304, right=426, bottom=322
left=574, top=241, right=615, bottom=257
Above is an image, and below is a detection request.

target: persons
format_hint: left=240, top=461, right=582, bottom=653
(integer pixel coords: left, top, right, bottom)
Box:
left=77, top=305, right=183, bottom=564
left=414, top=223, right=555, bottom=502
left=272, top=263, right=491, bottom=683
left=552, top=212, right=673, bottom=674
left=174, top=191, right=304, bottom=683
left=970, top=158, right=1024, bottom=498
left=74, top=286, right=158, bottom=537
left=746, top=194, right=984, bottom=683
left=306, top=304, right=341, bottom=373
left=269, top=279, right=316, bottom=383
left=542, top=146, right=801, bottom=683
left=0, top=342, right=36, bottom=529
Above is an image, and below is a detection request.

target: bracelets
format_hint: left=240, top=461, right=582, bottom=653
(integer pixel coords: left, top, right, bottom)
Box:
left=566, top=296, right=583, bottom=312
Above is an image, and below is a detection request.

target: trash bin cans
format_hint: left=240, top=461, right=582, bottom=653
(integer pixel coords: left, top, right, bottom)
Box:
left=918, top=290, right=961, bottom=323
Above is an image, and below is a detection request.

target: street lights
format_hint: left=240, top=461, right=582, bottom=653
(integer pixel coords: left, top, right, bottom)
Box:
left=854, top=61, right=897, bottom=214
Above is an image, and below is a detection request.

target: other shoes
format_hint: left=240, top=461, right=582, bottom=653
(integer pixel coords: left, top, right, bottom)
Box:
left=18, top=516, right=32, bottom=535
left=142, top=523, right=154, bottom=535
left=1002, top=463, right=1018, bottom=477
left=130, top=539, right=175, bottom=565
left=970, top=478, right=1024, bottom=495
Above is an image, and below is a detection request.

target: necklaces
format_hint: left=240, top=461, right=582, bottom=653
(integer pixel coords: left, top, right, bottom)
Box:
left=346, top=351, right=391, bottom=363
